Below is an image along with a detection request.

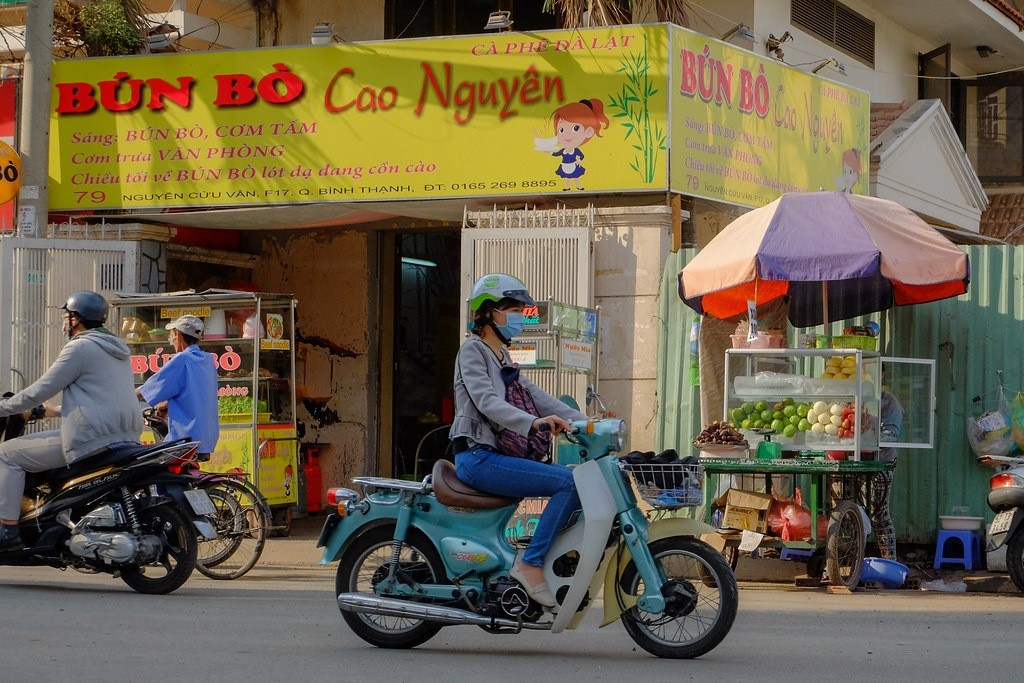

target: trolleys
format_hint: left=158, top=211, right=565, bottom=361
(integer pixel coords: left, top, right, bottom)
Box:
left=694, top=451, right=898, bottom=590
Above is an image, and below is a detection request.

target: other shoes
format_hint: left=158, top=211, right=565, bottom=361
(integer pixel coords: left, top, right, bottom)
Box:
left=510, top=562, right=556, bottom=608
left=0, top=522, right=23, bottom=552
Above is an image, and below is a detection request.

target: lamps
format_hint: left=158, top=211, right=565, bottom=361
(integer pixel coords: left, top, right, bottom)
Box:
left=310, top=22, right=346, bottom=45
left=720, top=21, right=758, bottom=43
left=976, top=46, right=997, bottom=58
left=148, top=34, right=177, bottom=53
left=812, top=57, right=846, bottom=76
left=484, top=10, right=514, bottom=32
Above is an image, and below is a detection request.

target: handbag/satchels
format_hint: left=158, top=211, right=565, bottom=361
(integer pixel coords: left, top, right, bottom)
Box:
left=965, top=384, right=1019, bottom=457
left=1009, top=391, right=1024, bottom=452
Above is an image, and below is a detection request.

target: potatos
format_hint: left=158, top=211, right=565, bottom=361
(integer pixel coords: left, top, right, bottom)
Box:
left=695, top=421, right=748, bottom=445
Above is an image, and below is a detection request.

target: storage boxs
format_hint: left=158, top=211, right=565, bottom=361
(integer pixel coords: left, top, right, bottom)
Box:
left=816, top=336, right=878, bottom=350
left=729, top=334, right=786, bottom=348
left=716, top=487, right=773, bottom=534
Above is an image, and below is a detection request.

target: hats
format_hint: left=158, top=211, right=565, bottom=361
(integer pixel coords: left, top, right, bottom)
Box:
left=165, top=314, right=205, bottom=340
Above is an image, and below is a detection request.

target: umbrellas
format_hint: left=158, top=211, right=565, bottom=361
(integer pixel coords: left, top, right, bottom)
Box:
left=677, top=188, right=972, bottom=349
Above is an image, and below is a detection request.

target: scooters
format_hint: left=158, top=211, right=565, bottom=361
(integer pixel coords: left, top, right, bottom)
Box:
left=315, top=383, right=740, bottom=661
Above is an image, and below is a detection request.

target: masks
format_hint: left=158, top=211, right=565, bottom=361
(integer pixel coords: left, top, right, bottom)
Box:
left=61, top=321, right=69, bottom=338
left=490, top=308, right=525, bottom=339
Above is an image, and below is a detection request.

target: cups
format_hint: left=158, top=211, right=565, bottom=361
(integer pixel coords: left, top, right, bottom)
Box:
left=815, top=335, right=832, bottom=348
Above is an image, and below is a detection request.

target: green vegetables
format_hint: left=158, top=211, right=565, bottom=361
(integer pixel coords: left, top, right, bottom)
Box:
left=217, top=395, right=268, bottom=414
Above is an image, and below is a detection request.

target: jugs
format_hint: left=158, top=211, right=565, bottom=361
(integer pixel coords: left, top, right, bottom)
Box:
left=204, top=309, right=226, bottom=340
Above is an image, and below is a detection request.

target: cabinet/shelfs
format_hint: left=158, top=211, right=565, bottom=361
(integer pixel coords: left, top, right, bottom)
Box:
left=724, top=348, right=937, bottom=465
left=110, top=293, right=299, bottom=538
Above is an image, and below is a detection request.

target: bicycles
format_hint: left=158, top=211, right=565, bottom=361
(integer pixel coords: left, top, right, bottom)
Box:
left=132, top=402, right=272, bottom=581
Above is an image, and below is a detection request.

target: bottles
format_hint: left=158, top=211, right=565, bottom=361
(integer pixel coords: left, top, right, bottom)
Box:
left=243, top=313, right=265, bottom=339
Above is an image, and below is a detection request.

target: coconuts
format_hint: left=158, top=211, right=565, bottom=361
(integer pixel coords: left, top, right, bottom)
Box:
left=728, top=397, right=811, bottom=437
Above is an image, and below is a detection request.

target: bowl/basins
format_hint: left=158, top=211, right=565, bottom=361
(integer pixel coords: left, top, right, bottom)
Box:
left=150, top=333, right=169, bottom=342
left=267, top=316, right=283, bottom=339
left=860, top=557, right=910, bottom=589
left=939, top=515, right=984, bottom=530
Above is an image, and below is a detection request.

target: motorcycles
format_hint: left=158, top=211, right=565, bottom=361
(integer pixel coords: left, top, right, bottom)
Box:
left=0, top=391, right=220, bottom=596
left=975, top=453, right=1024, bottom=594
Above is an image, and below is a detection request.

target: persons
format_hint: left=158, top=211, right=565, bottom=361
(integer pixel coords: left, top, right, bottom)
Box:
left=0, top=291, right=143, bottom=554
left=135, top=315, right=219, bottom=454
left=448, top=273, right=600, bottom=608
left=830, top=363, right=904, bottom=561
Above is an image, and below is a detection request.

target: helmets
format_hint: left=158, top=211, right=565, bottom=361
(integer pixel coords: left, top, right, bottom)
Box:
left=470, top=273, right=539, bottom=312
left=57, top=290, right=110, bottom=323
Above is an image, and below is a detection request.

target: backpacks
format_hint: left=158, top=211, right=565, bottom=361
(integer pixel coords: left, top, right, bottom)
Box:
left=458, top=339, right=554, bottom=465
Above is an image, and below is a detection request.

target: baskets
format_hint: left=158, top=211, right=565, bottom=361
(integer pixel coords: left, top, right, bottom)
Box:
left=729, top=335, right=787, bottom=348
left=816, top=334, right=878, bottom=351
left=620, top=463, right=705, bottom=506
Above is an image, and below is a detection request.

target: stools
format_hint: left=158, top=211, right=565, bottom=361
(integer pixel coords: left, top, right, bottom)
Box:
left=934, top=529, right=981, bottom=570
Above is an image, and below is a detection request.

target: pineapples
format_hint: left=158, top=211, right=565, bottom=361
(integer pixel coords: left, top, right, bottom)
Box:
left=819, top=355, right=870, bottom=381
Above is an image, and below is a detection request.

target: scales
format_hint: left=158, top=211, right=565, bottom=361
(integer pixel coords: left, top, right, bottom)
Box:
left=752, top=428, right=782, bottom=459
